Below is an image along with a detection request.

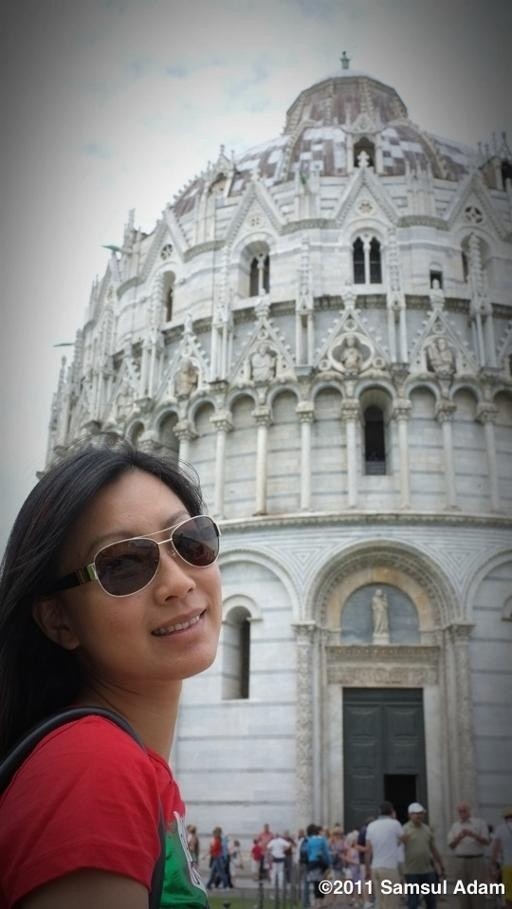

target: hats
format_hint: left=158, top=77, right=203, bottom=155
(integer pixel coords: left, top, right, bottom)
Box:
left=407, top=801, right=427, bottom=816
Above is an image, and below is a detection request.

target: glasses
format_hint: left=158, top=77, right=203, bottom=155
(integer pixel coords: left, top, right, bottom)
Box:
left=37, top=512, right=224, bottom=601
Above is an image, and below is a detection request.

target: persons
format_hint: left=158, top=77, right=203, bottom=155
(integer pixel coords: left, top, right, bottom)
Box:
left=430, top=339, right=453, bottom=371
left=371, top=589, right=389, bottom=634
left=0, top=442, right=222, bottom=909
left=339, top=335, right=362, bottom=375
left=251, top=343, right=275, bottom=379
left=175, top=361, right=198, bottom=396
